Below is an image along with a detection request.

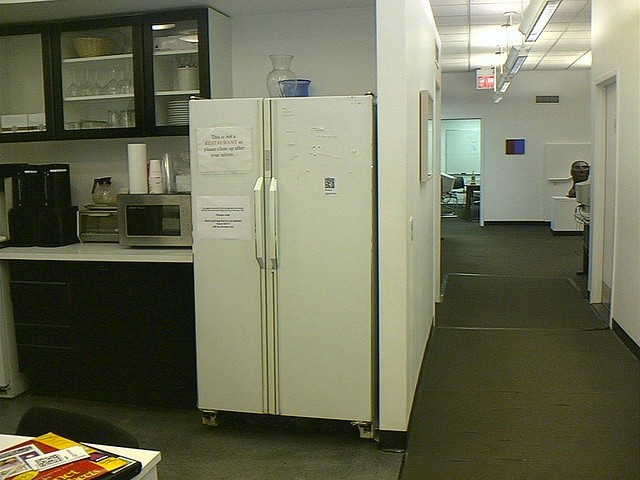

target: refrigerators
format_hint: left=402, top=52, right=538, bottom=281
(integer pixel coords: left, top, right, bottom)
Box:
left=187, top=90, right=379, bottom=442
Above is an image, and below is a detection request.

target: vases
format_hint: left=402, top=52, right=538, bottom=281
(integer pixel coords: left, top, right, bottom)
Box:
left=266, top=54, right=298, bottom=98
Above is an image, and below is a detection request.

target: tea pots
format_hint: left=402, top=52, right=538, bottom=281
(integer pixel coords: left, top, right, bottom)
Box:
left=91, top=176, right=118, bottom=206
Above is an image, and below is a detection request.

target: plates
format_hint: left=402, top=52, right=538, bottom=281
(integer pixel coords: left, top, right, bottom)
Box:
left=167, top=100, right=189, bottom=125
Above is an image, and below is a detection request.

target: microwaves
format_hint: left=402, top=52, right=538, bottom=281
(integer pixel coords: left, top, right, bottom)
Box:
left=116, top=194, right=193, bottom=249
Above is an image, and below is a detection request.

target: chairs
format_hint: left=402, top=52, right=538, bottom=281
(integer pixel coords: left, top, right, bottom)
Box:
left=17, top=407, right=140, bottom=449
left=447, top=176, right=468, bottom=209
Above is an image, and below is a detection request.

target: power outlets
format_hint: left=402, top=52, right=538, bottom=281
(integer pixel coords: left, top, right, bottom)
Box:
left=408, top=217, right=415, bottom=244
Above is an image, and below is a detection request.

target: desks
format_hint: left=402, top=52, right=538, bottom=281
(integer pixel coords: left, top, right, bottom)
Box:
left=0, top=433, right=161, bottom=480
left=465, top=183, right=481, bottom=220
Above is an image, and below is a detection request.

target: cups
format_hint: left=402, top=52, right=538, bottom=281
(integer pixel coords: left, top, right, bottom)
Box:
left=68, top=119, right=108, bottom=130
left=107, top=108, right=136, bottom=128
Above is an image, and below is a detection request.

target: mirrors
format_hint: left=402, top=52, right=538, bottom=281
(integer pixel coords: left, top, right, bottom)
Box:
left=420, top=90, right=434, bottom=183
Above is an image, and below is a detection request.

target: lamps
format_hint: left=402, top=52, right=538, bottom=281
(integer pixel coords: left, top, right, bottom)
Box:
left=517, top=0, right=563, bottom=42
left=490, top=92, right=503, bottom=104
left=505, top=46, right=528, bottom=75
left=498, top=76, right=510, bottom=93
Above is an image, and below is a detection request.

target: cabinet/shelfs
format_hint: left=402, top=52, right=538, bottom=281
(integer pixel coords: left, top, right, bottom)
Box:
left=0, top=22, right=55, bottom=143
left=1, top=248, right=196, bottom=412
left=53, top=7, right=232, bottom=140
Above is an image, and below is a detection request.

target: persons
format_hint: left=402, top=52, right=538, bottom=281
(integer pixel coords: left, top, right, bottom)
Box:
left=568, top=160, right=591, bottom=198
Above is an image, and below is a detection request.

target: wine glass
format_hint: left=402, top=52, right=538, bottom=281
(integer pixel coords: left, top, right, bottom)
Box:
left=66, top=63, right=134, bottom=98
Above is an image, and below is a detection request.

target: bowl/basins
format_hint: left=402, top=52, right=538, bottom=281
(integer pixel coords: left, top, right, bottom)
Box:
left=278, top=80, right=311, bottom=97
